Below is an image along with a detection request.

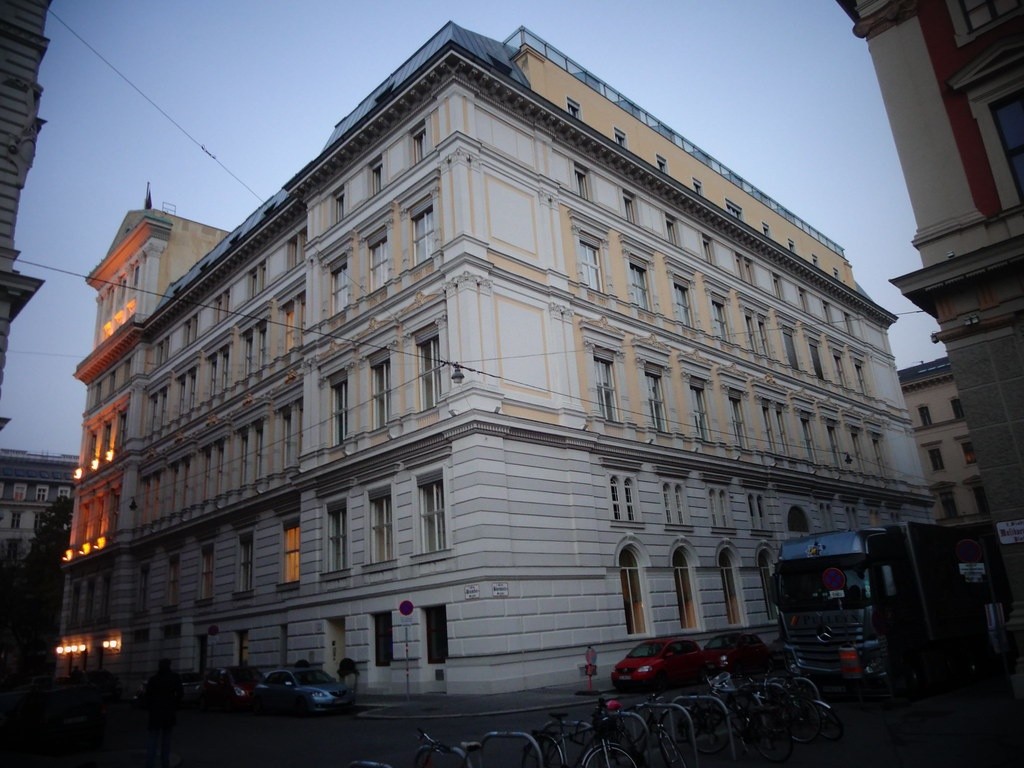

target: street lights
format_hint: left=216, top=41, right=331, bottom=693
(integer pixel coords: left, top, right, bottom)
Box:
left=56, top=639, right=119, bottom=654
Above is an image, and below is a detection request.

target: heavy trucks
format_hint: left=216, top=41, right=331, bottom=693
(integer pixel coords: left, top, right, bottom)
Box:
left=771, top=520, right=1015, bottom=705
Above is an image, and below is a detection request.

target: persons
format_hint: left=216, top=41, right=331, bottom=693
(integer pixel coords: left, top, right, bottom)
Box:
left=146, top=657, right=186, bottom=768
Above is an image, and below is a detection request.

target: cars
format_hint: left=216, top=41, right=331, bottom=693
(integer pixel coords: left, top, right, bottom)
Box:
left=255, top=666, right=354, bottom=713
left=203, top=666, right=251, bottom=710
left=611, top=637, right=703, bottom=692
left=702, top=633, right=771, bottom=678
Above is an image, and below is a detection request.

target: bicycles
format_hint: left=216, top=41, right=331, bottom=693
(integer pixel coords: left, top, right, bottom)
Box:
left=413, top=663, right=844, bottom=768
